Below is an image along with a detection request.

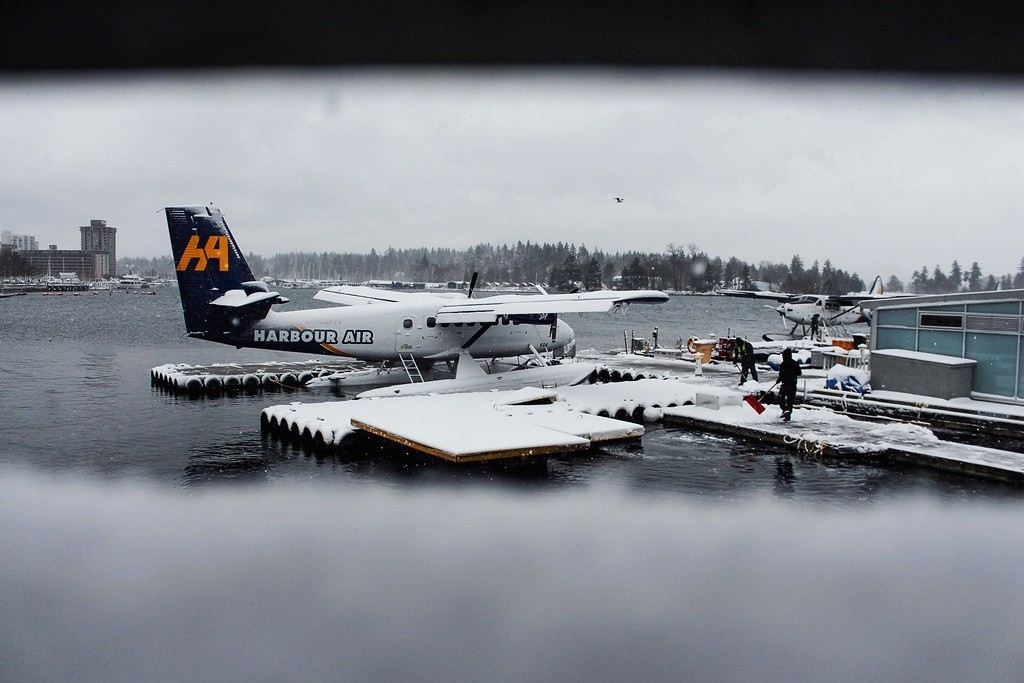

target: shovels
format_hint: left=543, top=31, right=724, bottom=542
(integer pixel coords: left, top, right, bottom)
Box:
left=744, top=383, right=778, bottom=414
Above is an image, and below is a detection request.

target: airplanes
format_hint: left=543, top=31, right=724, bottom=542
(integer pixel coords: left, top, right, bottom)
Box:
left=715, top=275, right=916, bottom=343
left=163, top=206, right=670, bottom=374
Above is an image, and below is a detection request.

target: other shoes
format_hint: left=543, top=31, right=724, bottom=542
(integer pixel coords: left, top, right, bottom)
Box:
left=783, top=418, right=791, bottom=423
left=780, top=410, right=790, bottom=418
left=739, top=383, right=743, bottom=386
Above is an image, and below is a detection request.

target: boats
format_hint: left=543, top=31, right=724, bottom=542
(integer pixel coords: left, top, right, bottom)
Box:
left=118, top=264, right=145, bottom=289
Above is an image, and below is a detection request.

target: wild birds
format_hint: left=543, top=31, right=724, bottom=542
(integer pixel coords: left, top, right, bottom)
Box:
left=614, top=197, right=623, bottom=203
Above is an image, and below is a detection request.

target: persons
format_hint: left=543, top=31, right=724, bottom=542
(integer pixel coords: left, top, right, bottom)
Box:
left=735, top=337, right=758, bottom=386
left=810, top=314, right=821, bottom=341
left=776, top=349, right=802, bottom=422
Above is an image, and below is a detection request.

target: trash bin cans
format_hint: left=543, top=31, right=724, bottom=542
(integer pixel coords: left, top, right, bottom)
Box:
left=632, top=337, right=647, bottom=351
left=852, top=333, right=866, bottom=350
left=693, top=340, right=716, bottom=363
